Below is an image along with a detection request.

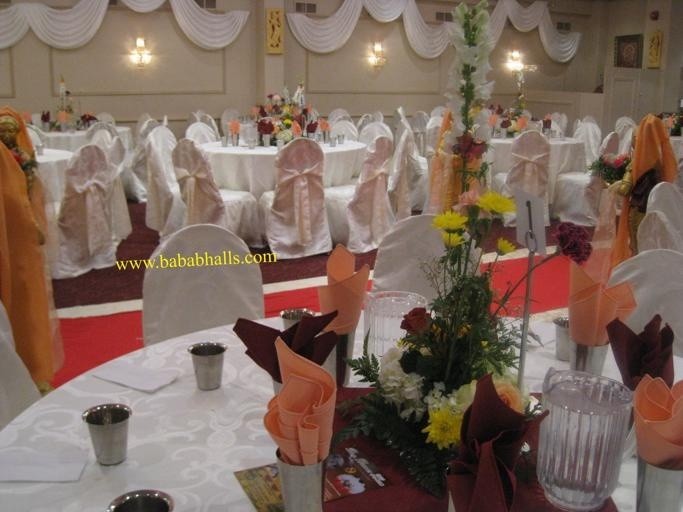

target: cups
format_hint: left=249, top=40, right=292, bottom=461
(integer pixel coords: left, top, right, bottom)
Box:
left=492, top=123, right=565, bottom=143
left=187, top=342, right=228, bottom=393
left=215, top=131, right=284, bottom=152
left=275, top=445, right=328, bottom=511
left=34, top=143, right=43, bottom=156
left=636, top=452, right=682, bottom=512
left=324, top=132, right=345, bottom=147
left=364, top=289, right=429, bottom=381
left=107, top=487, right=176, bottom=512
left=280, top=308, right=317, bottom=331
left=538, top=370, right=633, bottom=511
left=80, top=402, right=133, bottom=465
left=552, top=314, right=611, bottom=376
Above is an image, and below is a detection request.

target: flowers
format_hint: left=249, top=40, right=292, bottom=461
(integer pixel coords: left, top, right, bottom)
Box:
left=330, top=0, right=595, bottom=462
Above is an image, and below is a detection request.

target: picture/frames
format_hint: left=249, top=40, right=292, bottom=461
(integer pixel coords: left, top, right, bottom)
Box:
left=614, top=33, right=643, bottom=68
